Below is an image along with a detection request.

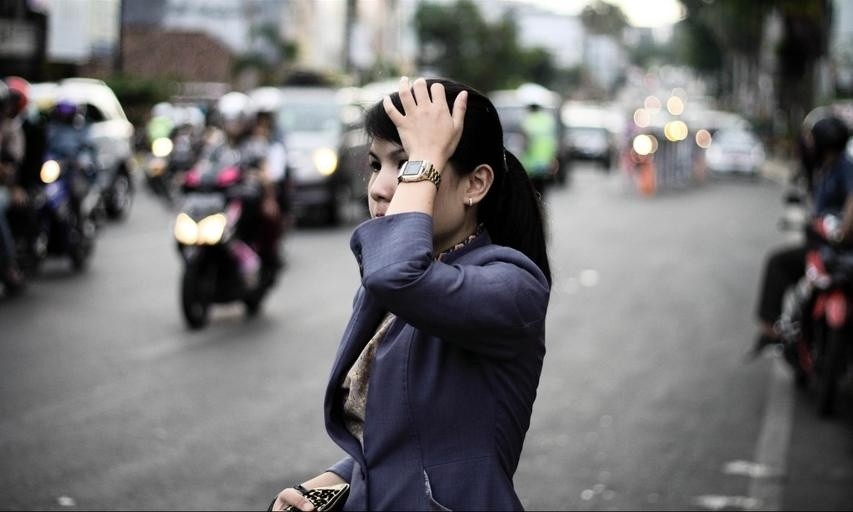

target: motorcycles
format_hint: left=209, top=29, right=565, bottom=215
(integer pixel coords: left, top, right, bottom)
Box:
left=171, top=149, right=296, bottom=328
left=754, top=188, right=851, bottom=418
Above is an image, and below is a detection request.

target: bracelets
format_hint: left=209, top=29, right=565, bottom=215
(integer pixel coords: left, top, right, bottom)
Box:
left=293, top=485, right=308, bottom=495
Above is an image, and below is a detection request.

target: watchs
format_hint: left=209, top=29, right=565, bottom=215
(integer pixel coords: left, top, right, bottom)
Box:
left=395, top=161, right=440, bottom=192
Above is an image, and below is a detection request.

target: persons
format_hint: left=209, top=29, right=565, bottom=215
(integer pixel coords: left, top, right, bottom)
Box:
left=512, top=86, right=558, bottom=199
left=0, top=65, right=291, bottom=293
left=268, top=73, right=551, bottom=511
left=751, top=99, right=851, bottom=362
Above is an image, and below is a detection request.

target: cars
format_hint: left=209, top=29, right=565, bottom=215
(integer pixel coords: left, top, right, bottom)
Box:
left=0, top=60, right=770, bottom=302
